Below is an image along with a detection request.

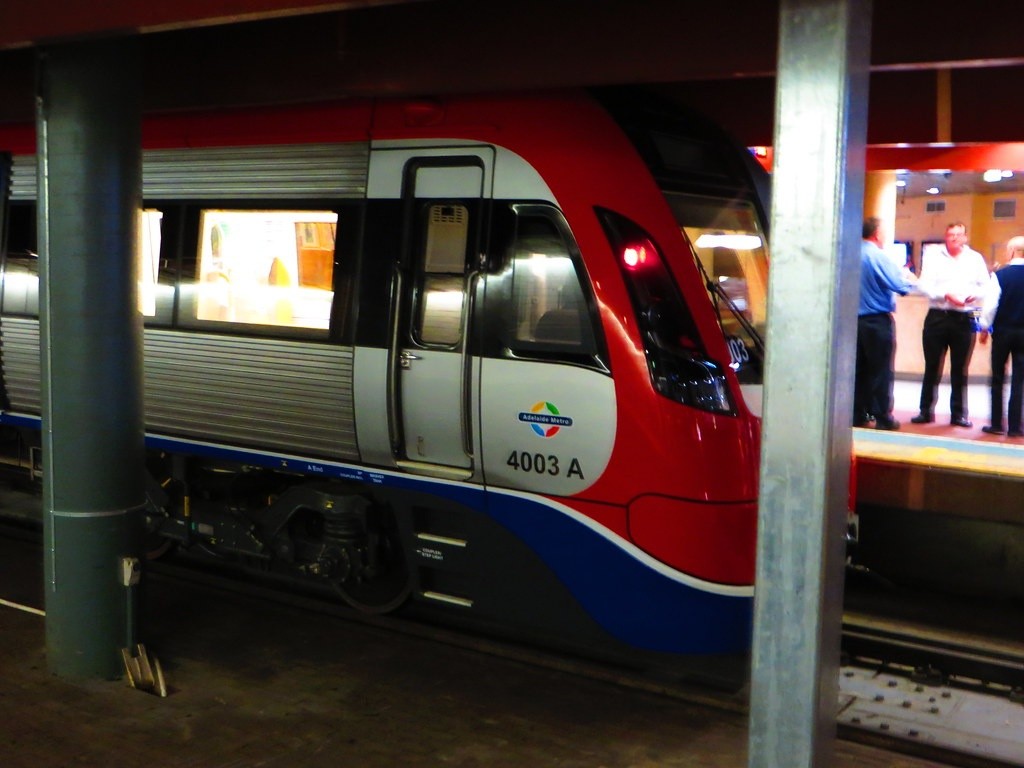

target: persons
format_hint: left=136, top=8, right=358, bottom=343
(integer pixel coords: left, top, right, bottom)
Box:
left=852, top=217, right=919, bottom=430
left=977, top=236, right=1024, bottom=438
left=910, top=223, right=991, bottom=428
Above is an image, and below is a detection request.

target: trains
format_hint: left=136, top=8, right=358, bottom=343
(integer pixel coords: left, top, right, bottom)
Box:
left=1, top=78, right=865, bottom=663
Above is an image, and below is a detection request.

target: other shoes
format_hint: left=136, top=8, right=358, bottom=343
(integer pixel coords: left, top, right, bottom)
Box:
left=912, top=414, right=936, bottom=423
left=1006, top=427, right=1023, bottom=437
left=951, top=419, right=972, bottom=428
left=873, top=420, right=900, bottom=430
left=854, top=408, right=876, bottom=425
left=981, top=424, right=1007, bottom=434
